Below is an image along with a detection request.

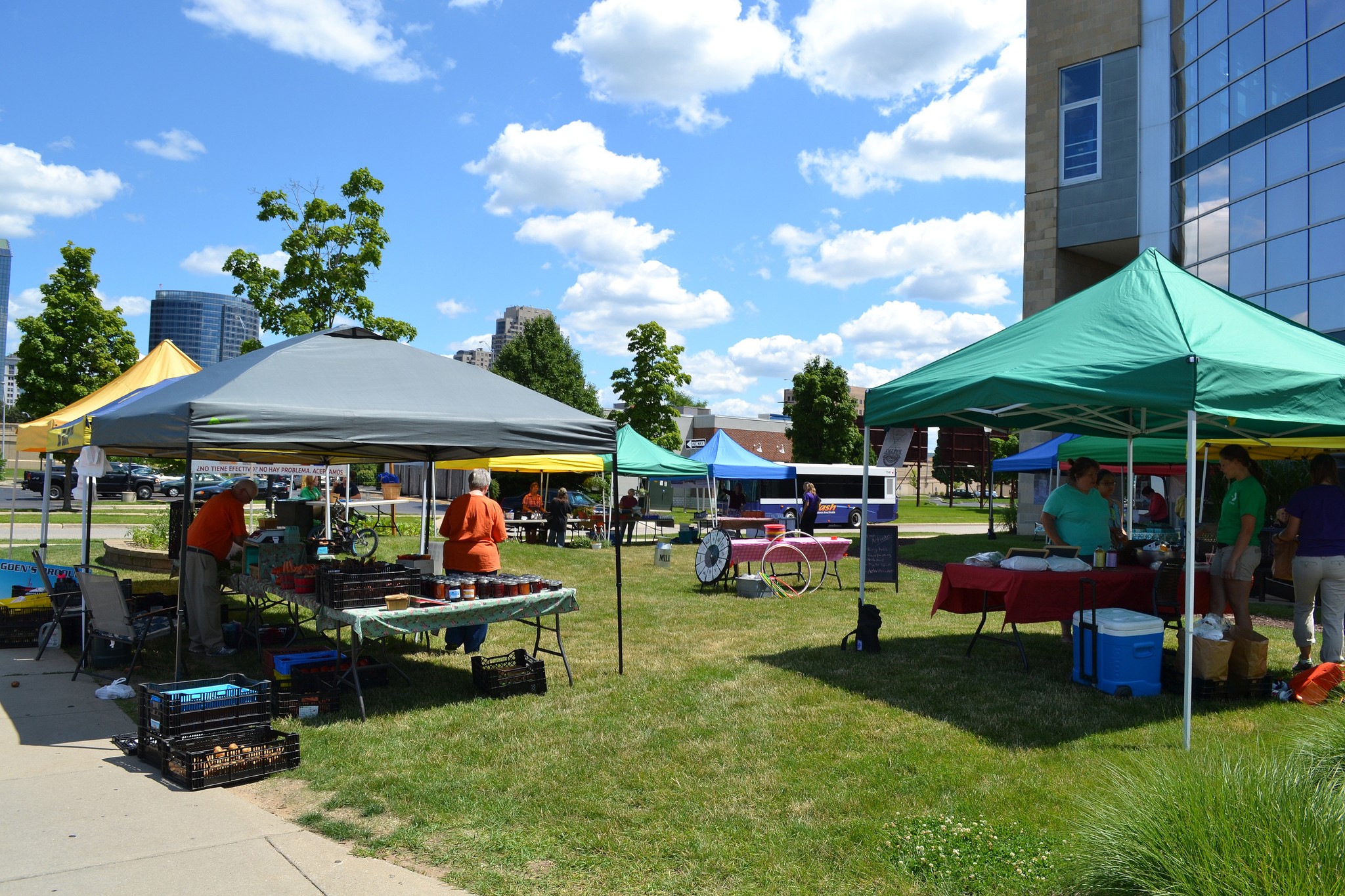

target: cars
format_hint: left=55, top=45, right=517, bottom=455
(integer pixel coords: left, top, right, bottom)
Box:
left=499, top=490, right=616, bottom=530
left=292, top=475, right=321, bottom=491
left=121, top=462, right=158, bottom=475
left=161, top=473, right=228, bottom=497
left=948, top=488, right=972, bottom=499
left=193, top=476, right=290, bottom=503
left=267, top=474, right=292, bottom=484
left=971, top=489, right=999, bottom=499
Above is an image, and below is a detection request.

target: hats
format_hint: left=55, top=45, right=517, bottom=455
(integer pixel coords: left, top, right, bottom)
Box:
left=559, top=488, right=568, bottom=495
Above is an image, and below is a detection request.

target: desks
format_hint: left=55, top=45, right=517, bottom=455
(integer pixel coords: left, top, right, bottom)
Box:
left=1132, top=532, right=1179, bottom=544
left=931, top=562, right=1253, bottom=670
left=306, top=499, right=411, bottom=536
left=169, top=560, right=579, bottom=722
left=505, top=519, right=675, bottom=544
left=690, top=517, right=797, bottom=538
left=729, top=537, right=853, bottom=590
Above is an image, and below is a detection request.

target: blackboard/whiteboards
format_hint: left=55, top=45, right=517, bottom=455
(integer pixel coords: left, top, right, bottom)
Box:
left=861, top=525, right=898, bottom=583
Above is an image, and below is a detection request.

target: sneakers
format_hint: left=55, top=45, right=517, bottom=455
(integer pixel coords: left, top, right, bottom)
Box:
left=189, top=647, right=206, bottom=652
left=207, top=647, right=237, bottom=656
left=1292, top=658, right=1314, bottom=671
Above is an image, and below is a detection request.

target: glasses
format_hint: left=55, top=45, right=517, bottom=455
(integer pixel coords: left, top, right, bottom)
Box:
left=1097, top=480, right=1118, bottom=488
left=239, top=489, right=253, bottom=501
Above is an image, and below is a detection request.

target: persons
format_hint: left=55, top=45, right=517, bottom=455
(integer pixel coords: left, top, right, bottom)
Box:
left=1140, top=486, right=1169, bottom=523
left=800, top=482, right=821, bottom=537
left=178, top=479, right=259, bottom=656
left=1042, top=457, right=1114, bottom=651
left=437, top=467, right=508, bottom=654
left=1272, top=455, right=1345, bottom=670
left=1208, top=444, right=1266, bottom=632
left=618, top=489, right=638, bottom=545
left=1175, top=494, right=1186, bottom=546
left=300, top=476, right=361, bottom=530
left=720, top=478, right=747, bottom=539
left=1095, top=467, right=1128, bottom=545
left=521, top=482, right=572, bottom=548
left=1261, top=501, right=1291, bottom=560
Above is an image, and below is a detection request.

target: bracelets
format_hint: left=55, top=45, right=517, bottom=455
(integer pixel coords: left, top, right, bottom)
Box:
left=1277, top=532, right=1282, bottom=540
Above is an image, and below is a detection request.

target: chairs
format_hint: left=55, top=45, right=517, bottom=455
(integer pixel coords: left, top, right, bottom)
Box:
left=1141, top=558, right=1185, bottom=657
left=32, top=551, right=189, bottom=685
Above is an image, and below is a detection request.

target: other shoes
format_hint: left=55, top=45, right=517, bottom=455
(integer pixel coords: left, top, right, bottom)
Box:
left=1061, top=635, right=1074, bottom=644
left=445, top=644, right=459, bottom=651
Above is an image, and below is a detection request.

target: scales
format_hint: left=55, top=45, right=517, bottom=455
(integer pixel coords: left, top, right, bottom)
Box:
left=247, top=527, right=285, bottom=543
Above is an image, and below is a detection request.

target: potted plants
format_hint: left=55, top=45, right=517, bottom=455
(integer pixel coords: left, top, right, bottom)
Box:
left=591, top=541, right=602, bottom=549
left=635, top=489, right=648, bottom=498
left=573, top=506, right=595, bottom=519
left=584, top=476, right=611, bottom=504
left=258, top=508, right=279, bottom=530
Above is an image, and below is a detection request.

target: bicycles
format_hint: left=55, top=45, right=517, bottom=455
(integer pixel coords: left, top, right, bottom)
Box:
left=309, top=495, right=379, bottom=558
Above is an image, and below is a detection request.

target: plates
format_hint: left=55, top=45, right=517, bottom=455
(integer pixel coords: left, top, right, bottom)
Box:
left=340, top=499, right=351, bottom=502
left=764, top=524, right=786, bottom=541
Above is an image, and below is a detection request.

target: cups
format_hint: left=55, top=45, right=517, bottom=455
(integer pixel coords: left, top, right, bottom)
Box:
left=1205, top=553, right=1216, bottom=567
left=365, top=493, right=371, bottom=500
left=337, top=480, right=341, bottom=487
left=270, top=573, right=276, bottom=582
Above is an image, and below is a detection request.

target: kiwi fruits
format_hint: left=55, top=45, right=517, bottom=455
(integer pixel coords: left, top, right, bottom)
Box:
left=169, top=743, right=284, bottom=778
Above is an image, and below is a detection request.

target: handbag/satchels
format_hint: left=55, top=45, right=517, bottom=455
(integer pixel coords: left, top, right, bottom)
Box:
left=1292, top=661, right=1345, bottom=706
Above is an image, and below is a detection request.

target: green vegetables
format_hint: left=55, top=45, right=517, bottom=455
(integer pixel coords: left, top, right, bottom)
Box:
left=216, top=551, right=243, bottom=577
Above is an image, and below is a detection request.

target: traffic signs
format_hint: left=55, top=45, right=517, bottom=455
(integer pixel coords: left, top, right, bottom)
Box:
left=684, top=439, right=706, bottom=450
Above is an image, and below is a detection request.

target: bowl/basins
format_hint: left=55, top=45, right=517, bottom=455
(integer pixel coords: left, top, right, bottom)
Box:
left=506, top=512, right=514, bottom=519
left=831, top=536, right=839, bottom=540
left=1131, top=545, right=1181, bottom=565
left=330, top=494, right=340, bottom=503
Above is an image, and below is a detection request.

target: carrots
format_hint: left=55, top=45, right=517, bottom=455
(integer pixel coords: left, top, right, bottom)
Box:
left=280, top=691, right=330, bottom=711
left=283, top=560, right=297, bottom=572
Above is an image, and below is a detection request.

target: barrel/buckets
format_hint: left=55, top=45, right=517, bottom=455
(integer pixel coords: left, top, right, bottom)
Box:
left=280, top=573, right=295, bottom=589
left=381, top=483, right=401, bottom=500
left=294, top=574, right=315, bottom=594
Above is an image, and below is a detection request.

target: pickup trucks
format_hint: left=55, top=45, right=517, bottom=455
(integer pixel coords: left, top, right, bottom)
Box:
left=16, top=463, right=162, bottom=500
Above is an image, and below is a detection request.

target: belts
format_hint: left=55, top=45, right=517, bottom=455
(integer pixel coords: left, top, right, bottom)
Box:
left=188, top=547, right=213, bottom=555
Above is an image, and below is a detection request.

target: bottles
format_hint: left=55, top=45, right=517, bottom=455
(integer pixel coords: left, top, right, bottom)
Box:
left=38, top=622, right=62, bottom=650
left=1271, top=689, right=1289, bottom=701
left=1093, top=546, right=1105, bottom=569
left=1105, top=546, right=1117, bottom=569
left=1143, top=541, right=1183, bottom=551
left=654, top=536, right=672, bottom=568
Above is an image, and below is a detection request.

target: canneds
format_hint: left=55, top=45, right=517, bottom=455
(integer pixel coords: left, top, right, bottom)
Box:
left=418, top=572, right=562, bottom=602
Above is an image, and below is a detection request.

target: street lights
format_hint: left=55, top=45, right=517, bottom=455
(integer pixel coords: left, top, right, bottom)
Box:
left=0, top=381, right=7, bottom=462
left=479, top=340, right=494, bottom=370
left=984, top=426, right=998, bottom=540
left=234, top=315, right=246, bottom=352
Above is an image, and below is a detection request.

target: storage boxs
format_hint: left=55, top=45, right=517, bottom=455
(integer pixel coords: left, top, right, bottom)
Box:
left=1072, top=577, right=1164, bottom=699
left=0, top=580, right=81, bottom=649
left=589, top=514, right=673, bottom=519
left=315, top=566, right=422, bottom=610
left=139, top=645, right=388, bottom=792
left=735, top=576, right=774, bottom=598
left=1132, top=524, right=1175, bottom=533
left=471, top=648, right=548, bottom=700
left=678, top=531, right=699, bottom=544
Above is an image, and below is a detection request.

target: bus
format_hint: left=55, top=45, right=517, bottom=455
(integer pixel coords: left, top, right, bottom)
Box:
left=716, top=460, right=901, bottom=529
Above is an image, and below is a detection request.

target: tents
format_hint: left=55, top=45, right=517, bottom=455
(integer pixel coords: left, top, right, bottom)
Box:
left=595, top=423, right=715, bottom=547
left=988, top=432, right=1083, bottom=543
left=7, top=338, right=331, bottom=565
left=1056, top=436, right=1221, bottom=489
left=81, top=327, right=624, bottom=676
left=1184, top=437, right=1345, bottom=524
left=1058, top=461, right=1214, bottom=522
left=645, top=427, right=799, bottom=542
left=429, top=454, right=608, bottom=541
left=856, top=245, right=1345, bottom=752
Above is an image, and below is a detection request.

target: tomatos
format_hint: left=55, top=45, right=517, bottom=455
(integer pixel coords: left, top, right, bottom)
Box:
left=272, top=564, right=319, bottom=585
left=300, top=658, right=369, bottom=674
